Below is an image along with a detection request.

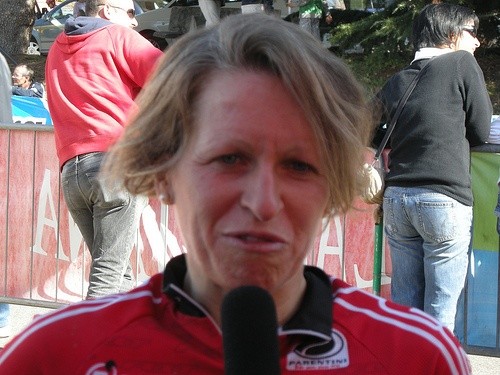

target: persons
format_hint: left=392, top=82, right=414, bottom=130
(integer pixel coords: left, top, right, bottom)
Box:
left=365, top=3, right=493, bottom=335
left=0, top=14, right=473, bottom=375
left=73, top=0, right=87, bottom=16
left=45, top=0, right=165, bottom=302
left=43, top=0, right=62, bottom=18
left=198, top=0, right=223, bottom=28
left=282, top=0, right=347, bottom=42
left=11, top=63, right=45, bottom=98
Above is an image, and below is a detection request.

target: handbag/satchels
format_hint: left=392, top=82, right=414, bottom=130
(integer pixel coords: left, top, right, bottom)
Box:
left=359, top=163, right=388, bottom=205
left=485, top=115, right=500, bottom=144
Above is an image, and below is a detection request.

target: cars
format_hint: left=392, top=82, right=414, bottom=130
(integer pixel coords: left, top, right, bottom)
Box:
left=134, top=0, right=208, bottom=51
left=24, top=0, right=88, bottom=56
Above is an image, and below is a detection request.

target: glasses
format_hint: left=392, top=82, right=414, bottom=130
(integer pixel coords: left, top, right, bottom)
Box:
left=97, top=3, right=136, bottom=19
left=13, top=72, right=29, bottom=77
left=462, top=28, right=477, bottom=38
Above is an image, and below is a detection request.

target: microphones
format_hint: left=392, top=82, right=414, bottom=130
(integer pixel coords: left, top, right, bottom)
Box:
left=220, top=286, right=281, bottom=375
